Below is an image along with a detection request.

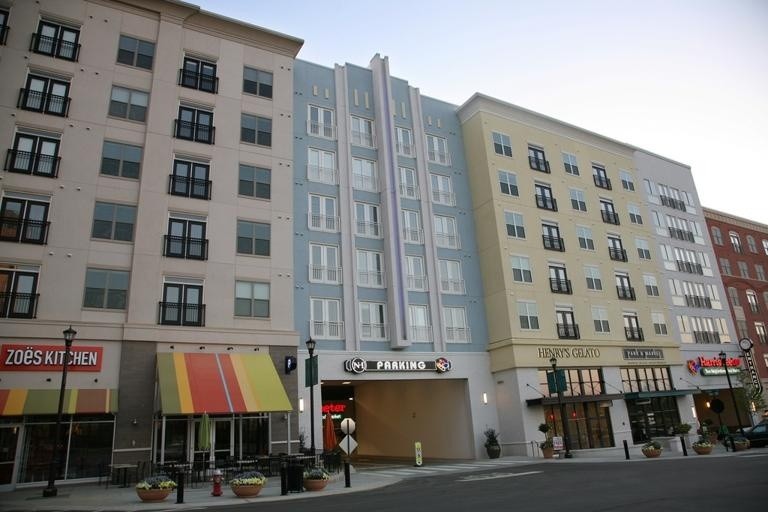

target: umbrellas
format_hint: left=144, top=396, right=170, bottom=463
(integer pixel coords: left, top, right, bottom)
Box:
left=322, top=407, right=338, bottom=471
left=197, top=410, right=212, bottom=481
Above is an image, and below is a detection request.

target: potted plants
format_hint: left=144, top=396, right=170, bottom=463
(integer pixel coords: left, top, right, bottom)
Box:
left=538, top=424, right=553, bottom=458
left=673, top=424, right=691, bottom=438
left=484, top=427, right=501, bottom=458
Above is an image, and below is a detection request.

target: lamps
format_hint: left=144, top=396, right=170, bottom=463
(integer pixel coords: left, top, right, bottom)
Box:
left=298, top=397, right=304, bottom=413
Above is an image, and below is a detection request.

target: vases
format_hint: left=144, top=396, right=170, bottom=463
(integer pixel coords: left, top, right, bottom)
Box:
left=231, top=484, right=262, bottom=498
left=642, top=449, right=662, bottom=458
left=730, top=443, right=747, bottom=450
left=692, top=446, right=712, bottom=455
left=136, top=488, right=172, bottom=502
left=302, top=480, right=329, bottom=491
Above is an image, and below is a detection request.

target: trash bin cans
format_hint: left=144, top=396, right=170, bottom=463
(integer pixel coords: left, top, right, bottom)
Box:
left=281, top=453, right=305, bottom=493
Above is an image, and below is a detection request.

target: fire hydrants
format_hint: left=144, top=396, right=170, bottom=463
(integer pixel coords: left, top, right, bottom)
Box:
left=210, top=469, right=225, bottom=497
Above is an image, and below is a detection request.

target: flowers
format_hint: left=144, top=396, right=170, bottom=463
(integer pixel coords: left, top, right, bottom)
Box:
left=642, top=441, right=663, bottom=449
left=691, top=439, right=713, bottom=446
left=727, top=437, right=750, bottom=443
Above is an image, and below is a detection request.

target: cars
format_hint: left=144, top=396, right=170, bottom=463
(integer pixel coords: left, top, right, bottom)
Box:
left=722, top=424, right=768, bottom=448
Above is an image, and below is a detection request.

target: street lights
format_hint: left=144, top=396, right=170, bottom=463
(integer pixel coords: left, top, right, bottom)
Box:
left=549, top=357, right=572, bottom=458
left=718, top=350, right=745, bottom=436
left=43, top=323, right=77, bottom=498
left=305, top=336, right=318, bottom=467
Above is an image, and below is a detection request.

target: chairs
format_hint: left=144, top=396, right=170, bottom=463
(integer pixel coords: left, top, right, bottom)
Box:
left=98, top=447, right=342, bottom=489
